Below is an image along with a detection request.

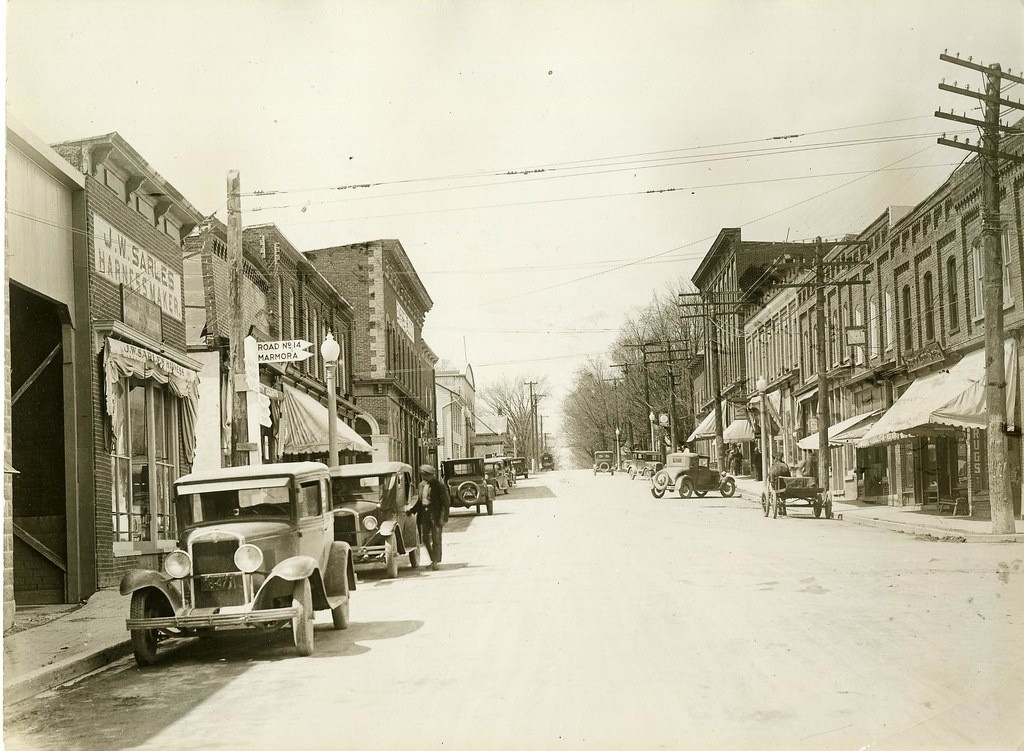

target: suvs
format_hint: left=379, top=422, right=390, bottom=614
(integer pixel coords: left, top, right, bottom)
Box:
left=538, top=453, right=555, bottom=472
left=440, top=457, right=495, bottom=515
left=650, top=452, right=736, bottom=499
left=330, top=461, right=422, bottom=580
left=485, top=456, right=528, bottom=494
left=119, top=460, right=358, bottom=668
left=593, top=451, right=614, bottom=476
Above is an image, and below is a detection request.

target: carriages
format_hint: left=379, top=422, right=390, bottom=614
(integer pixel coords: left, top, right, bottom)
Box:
left=761, top=452, right=833, bottom=519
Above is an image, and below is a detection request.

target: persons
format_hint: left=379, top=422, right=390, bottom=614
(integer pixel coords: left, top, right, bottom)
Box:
left=681, top=445, right=690, bottom=453
left=753, top=447, right=762, bottom=480
left=406, top=464, right=451, bottom=570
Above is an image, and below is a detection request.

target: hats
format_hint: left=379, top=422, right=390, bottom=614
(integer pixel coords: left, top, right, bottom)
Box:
left=419, top=464, right=435, bottom=477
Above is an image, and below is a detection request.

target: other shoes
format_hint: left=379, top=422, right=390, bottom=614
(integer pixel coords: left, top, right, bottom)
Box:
left=425, top=563, right=440, bottom=571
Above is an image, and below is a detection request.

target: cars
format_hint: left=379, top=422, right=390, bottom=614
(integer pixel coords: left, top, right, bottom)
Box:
left=623, top=451, right=664, bottom=480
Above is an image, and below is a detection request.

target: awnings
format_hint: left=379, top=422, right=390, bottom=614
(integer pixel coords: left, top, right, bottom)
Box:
left=686, top=402, right=727, bottom=443
left=712, top=421, right=756, bottom=444
left=281, top=384, right=377, bottom=455
left=858, top=338, right=1017, bottom=449
left=798, top=409, right=884, bottom=451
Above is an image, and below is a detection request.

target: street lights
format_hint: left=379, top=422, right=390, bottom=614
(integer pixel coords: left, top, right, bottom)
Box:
left=756, top=376, right=767, bottom=490
left=513, top=434, right=517, bottom=458
left=464, top=407, right=471, bottom=457
left=615, top=427, right=621, bottom=471
left=320, top=327, right=340, bottom=466
left=649, top=412, right=655, bottom=449
